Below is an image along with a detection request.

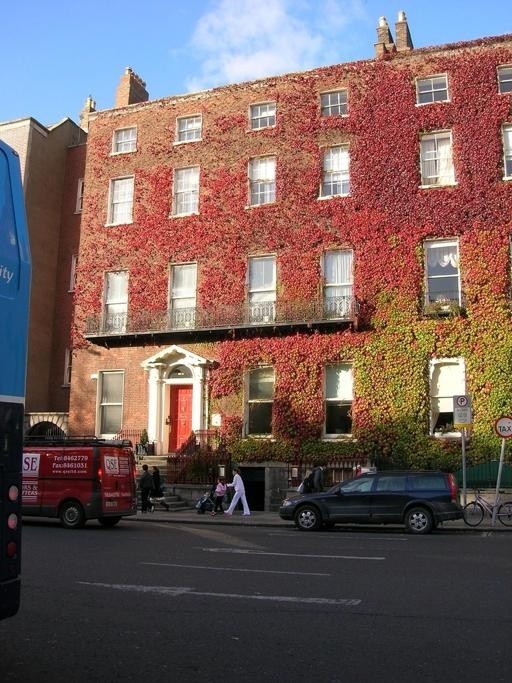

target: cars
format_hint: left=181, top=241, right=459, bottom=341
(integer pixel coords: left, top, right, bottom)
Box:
left=277, top=467, right=466, bottom=534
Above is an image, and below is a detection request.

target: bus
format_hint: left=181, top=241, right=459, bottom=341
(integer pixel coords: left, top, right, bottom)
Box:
left=1, top=141, right=32, bottom=620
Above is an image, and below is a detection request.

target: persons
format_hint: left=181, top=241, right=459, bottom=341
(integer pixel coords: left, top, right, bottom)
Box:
left=211, top=476, right=226, bottom=516
left=137, top=464, right=169, bottom=513
left=224, top=468, right=250, bottom=515
left=297, top=462, right=327, bottom=494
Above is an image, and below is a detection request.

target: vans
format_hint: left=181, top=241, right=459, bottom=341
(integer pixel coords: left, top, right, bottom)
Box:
left=22, top=431, right=139, bottom=530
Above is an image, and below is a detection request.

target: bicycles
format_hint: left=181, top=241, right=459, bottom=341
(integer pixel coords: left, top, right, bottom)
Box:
left=461, top=485, right=512, bottom=528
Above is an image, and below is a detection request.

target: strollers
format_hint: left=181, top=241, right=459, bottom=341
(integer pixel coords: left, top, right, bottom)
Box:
left=195, top=482, right=225, bottom=516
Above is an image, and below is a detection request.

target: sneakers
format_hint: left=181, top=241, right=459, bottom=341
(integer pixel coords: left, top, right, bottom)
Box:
left=151, top=505, right=155, bottom=512
left=224, top=510, right=232, bottom=516
left=165, top=503, right=170, bottom=511
left=242, top=512, right=250, bottom=515
